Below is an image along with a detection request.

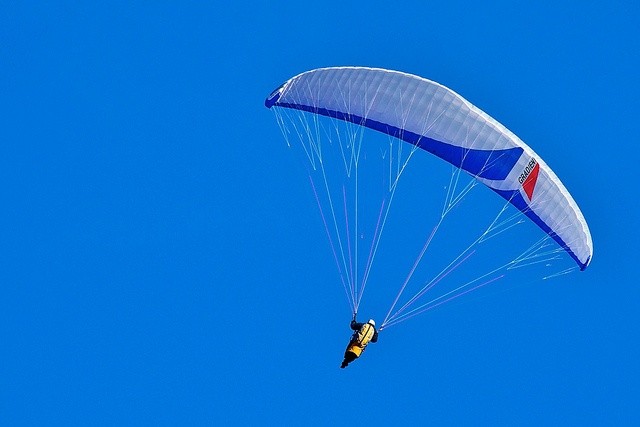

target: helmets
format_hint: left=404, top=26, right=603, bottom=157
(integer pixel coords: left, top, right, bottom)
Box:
left=369, top=319, right=375, bottom=326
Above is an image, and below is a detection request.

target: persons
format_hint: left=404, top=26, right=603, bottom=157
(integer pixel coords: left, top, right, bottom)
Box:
left=340, top=315, right=381, bottom=369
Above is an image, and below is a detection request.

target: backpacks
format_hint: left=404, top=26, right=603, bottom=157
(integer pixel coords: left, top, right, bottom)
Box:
left=356, top=323, right=375, bottom=348
